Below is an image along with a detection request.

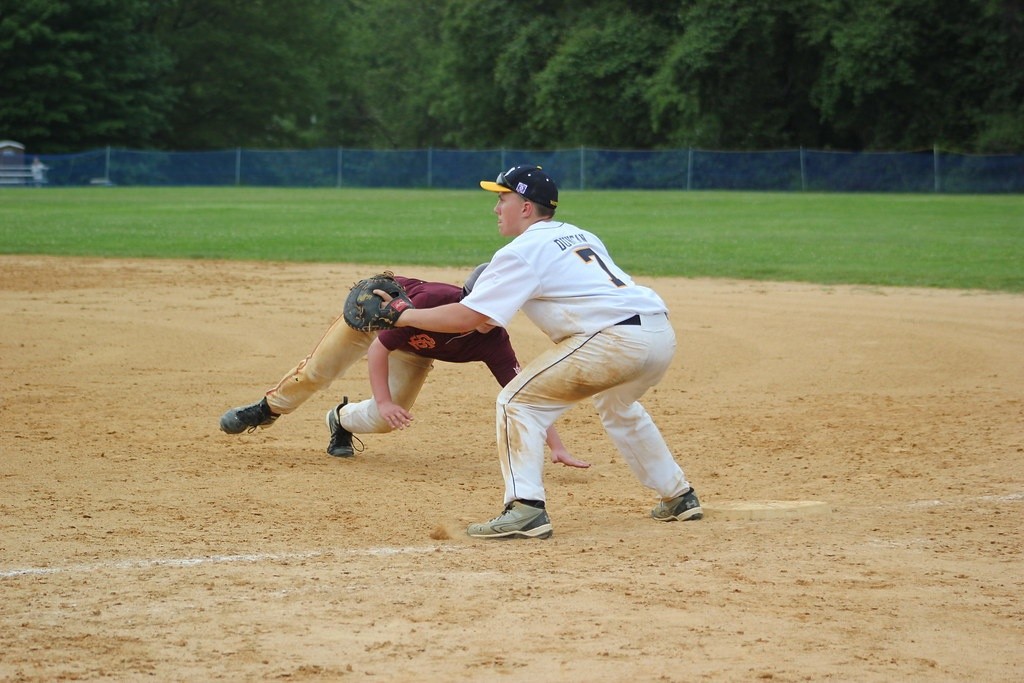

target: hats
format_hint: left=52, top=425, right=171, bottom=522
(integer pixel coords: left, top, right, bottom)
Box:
left=464, top=262, right=489, bottom=295
left=480, top=166, right=558, bottom=209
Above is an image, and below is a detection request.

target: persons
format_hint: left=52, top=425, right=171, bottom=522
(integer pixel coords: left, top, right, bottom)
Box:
left=220, top=262, right=592, bottom=468
left=372, top=164, right=704, bottom=544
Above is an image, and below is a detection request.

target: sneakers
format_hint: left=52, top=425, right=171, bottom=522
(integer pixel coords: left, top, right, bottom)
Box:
left=467, top=501, right=552, bottom=540
left=220, top=396, right=281, bottom=434
left=651, top=487, right=703, bottom=521
left=326, top=396, right=364, bottom=458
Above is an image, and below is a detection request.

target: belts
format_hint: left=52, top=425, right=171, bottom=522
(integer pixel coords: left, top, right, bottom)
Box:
left=614, top=312, right=669, bottom=325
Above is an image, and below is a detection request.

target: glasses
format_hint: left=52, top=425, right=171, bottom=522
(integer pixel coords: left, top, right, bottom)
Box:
left=496, top=172, right=527, bottom=201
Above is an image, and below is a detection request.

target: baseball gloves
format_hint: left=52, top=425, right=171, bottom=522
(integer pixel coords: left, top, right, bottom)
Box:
left=343, top=274, right=417, bottom=334
